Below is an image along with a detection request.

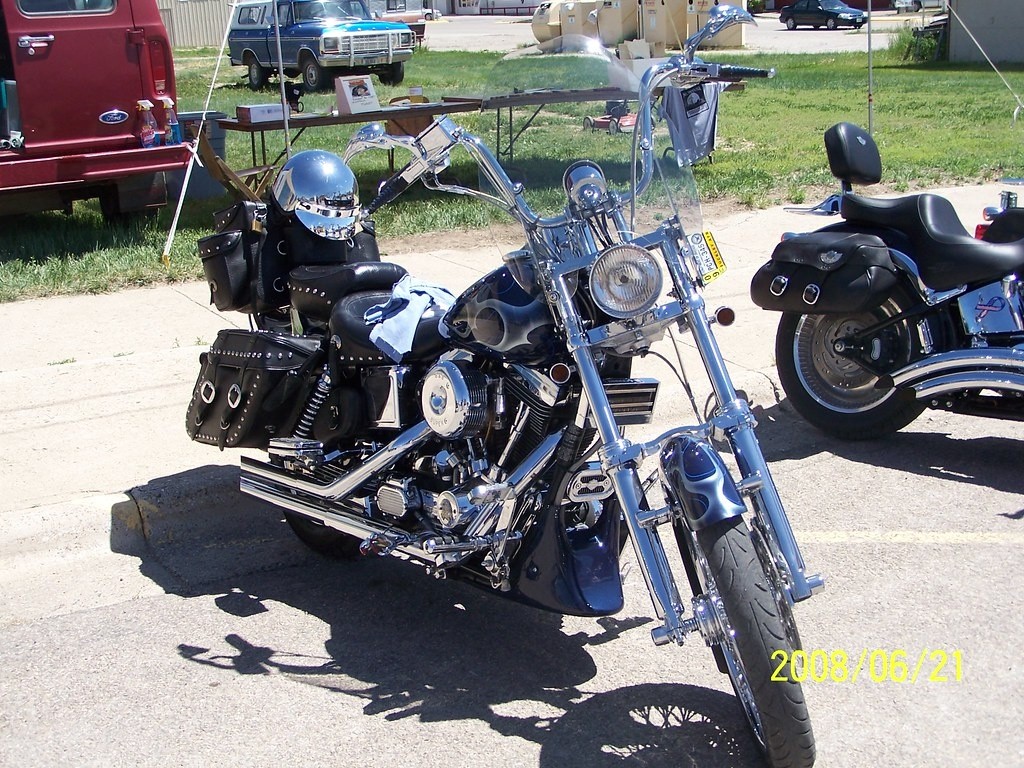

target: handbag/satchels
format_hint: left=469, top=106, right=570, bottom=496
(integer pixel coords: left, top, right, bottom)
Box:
left=749, top=231, right=899, bottom=317
left=184, top=325, right=327, bottom=453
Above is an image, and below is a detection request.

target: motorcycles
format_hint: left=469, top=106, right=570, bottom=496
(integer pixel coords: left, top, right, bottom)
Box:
left=185, top=5, right=823, bottom=767
left=752, top=121, right=1024, bottom=441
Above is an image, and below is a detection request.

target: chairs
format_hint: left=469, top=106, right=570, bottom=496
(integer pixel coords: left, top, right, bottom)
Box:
left=384, top=96, right=434, bottom=178
left=189, top=123, right=282, bottom=203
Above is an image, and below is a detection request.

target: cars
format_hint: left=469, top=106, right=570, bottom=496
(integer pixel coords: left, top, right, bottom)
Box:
left=906, top=0, right=948, bottom=13
left=396, top=4, right=441, bottom=23
left=779, top=0, right=868, bottom=30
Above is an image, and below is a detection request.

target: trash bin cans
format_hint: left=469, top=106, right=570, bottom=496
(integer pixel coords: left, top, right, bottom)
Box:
left=164, top=110, right=232, bottom=203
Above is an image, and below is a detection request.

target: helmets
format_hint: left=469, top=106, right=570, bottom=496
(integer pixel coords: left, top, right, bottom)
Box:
left=270, top=149, right=361, bottom=241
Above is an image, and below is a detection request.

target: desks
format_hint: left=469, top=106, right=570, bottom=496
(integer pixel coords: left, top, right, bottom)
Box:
left=216, top=79, right=748, bottom=196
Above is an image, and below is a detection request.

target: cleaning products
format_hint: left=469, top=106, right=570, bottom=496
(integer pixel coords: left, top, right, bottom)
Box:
left=137, top=100, right=160, bottom=148
left=155, top=96, right=182, bottom=146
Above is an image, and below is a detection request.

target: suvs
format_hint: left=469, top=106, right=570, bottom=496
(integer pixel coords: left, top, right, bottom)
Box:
left=228, top=0, right=414, bottom=91
left=0, top=0, right=193, bottom=235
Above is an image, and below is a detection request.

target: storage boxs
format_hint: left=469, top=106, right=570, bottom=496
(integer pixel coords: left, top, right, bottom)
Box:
left=560, top=0, right=748, bottom=49
left=197, top=201, right=382, bottom=315
left=236, top=104, right=291, bottom=124
